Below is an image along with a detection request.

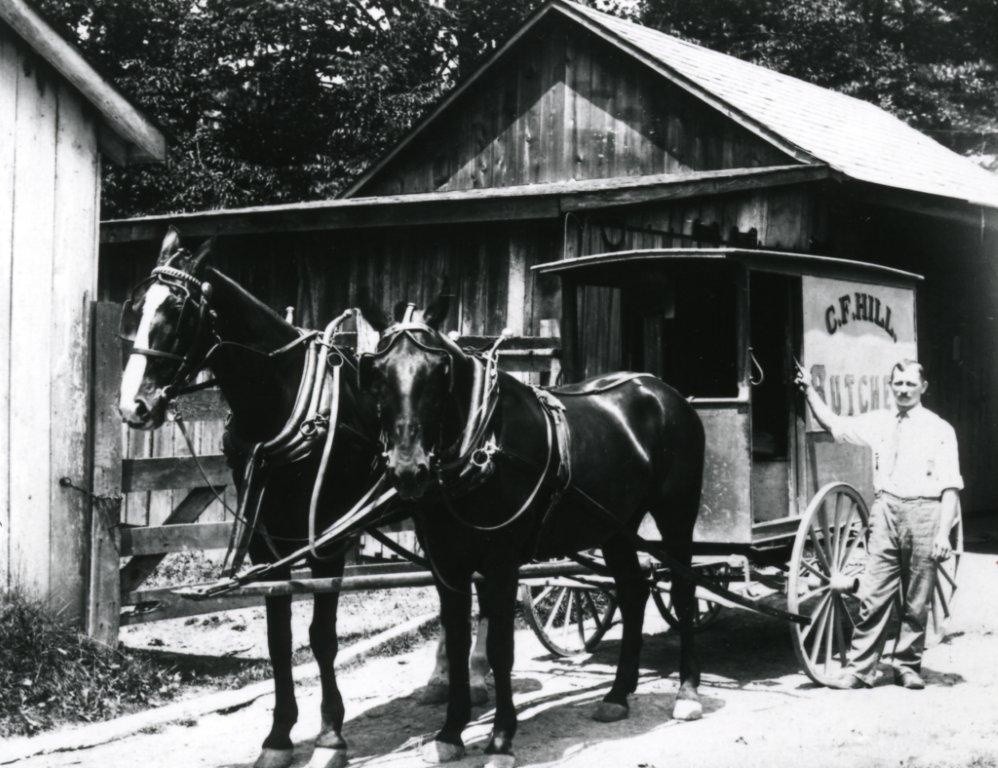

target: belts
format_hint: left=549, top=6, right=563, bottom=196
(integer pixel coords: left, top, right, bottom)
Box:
left=874, top=491, right=942, bottom=505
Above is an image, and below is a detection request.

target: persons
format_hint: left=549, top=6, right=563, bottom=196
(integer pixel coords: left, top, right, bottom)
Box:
left=794, top=358, right=964, bottom=692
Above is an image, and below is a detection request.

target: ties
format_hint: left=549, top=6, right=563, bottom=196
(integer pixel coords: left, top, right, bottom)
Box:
left=885, top=413, right=906, bottom=480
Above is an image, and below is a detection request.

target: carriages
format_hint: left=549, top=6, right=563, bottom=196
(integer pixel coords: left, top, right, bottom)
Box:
left=109, top=224, right=964, bottom=767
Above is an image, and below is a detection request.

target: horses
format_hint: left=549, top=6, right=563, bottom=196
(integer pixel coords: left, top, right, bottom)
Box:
left=351, top=287, right=707, bottom=768
left=115, top=224, right=492, bottom=768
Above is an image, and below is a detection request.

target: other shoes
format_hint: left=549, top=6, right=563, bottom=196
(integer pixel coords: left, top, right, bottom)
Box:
left=825, top=674, right=865, bottom=689
left=895, top=671, right=924, bottom=689
